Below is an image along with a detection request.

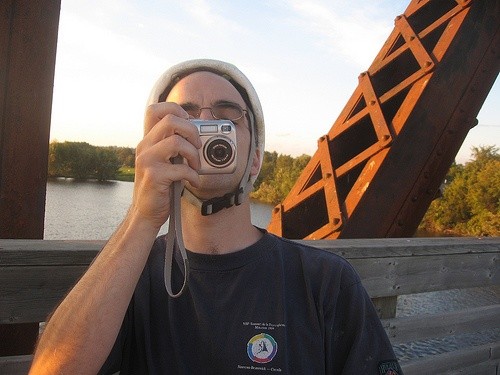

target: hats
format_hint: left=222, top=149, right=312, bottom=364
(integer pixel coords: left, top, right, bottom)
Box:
left=147, top=59, right=265, bottom=184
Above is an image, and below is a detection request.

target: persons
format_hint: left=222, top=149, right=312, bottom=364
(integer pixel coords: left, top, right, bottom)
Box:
left=27, top=58, right=406, bottom=375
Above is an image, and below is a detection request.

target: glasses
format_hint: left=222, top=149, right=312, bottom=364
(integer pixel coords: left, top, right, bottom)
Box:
left=185, top=102, right=249, bottom=122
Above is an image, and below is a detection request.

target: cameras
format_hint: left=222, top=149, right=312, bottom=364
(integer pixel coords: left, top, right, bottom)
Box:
left=176, top=119, right=236, bottom=174
